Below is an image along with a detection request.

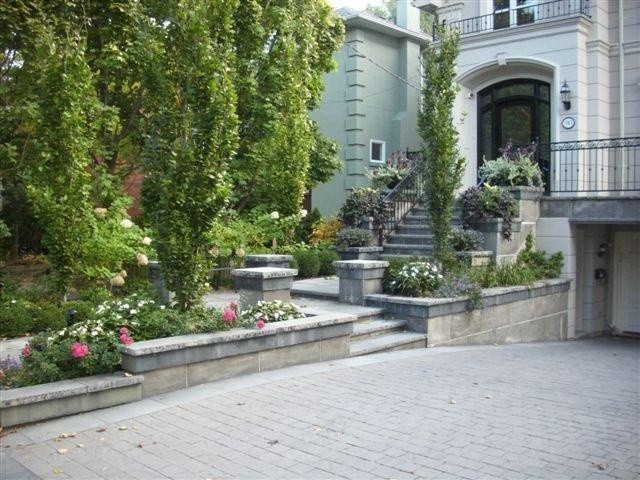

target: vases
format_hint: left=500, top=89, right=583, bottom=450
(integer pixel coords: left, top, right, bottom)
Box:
left=386, top=181, right=398, bottom=190
left=454, top=244, right=464, bottom=253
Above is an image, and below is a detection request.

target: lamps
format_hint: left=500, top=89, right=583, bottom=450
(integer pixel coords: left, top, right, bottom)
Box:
left=595, top=237, right=612, bottom=258
left=560, top=79, right=571, bottom=111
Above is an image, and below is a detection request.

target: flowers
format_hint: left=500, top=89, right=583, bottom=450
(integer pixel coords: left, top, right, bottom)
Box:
left=445, top=228, right=487, bottom=252
left=363, top=147, right=422, bottom=190
